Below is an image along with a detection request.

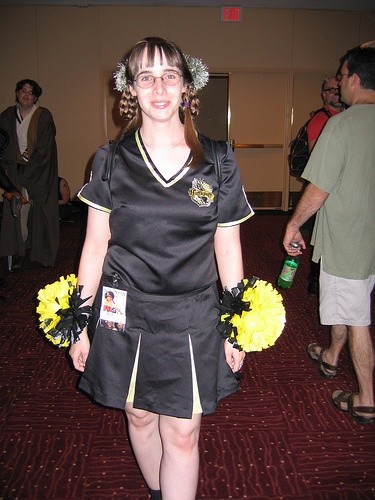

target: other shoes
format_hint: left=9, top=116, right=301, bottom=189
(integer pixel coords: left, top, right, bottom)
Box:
left=0, top=296, right=5, bottom=302
left=308, top=272, right=319, bottom=297
left=0, top=276, right=6, bottom=286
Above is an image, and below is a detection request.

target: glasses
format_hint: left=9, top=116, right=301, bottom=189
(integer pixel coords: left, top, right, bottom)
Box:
left=337, top=73, right=356, bottom=80
left=323, top=88, right=339, bottom=93
left=131, top=71, right=184, bottom=89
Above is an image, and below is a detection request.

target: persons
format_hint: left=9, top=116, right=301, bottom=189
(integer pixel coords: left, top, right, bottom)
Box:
left=58, top=177, right=83, bottom=221
left=68, top=36, right=255, bottom=500
left=1, top=79, right=59, bottom=269
left=282, top=46, right=375, bottom=423
left=306, top=76, right=347, bottom=297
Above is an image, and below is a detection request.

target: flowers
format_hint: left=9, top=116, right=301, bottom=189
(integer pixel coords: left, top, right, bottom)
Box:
left=181, top=51, right=210, bottom=92
left=110, top=59, right=130, bottom=92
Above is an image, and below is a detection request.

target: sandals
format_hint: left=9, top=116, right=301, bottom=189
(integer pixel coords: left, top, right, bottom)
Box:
left=308, top=342, right=339, bottom=378
left=331, top=389, right=375, bottom=425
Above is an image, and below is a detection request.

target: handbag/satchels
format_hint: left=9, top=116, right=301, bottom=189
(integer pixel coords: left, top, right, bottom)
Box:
left=287, top=108, right=332, bottom=182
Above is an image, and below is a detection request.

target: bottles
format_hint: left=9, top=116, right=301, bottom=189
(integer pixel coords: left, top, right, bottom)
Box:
left=278, top=243, right=300, bottom=289
